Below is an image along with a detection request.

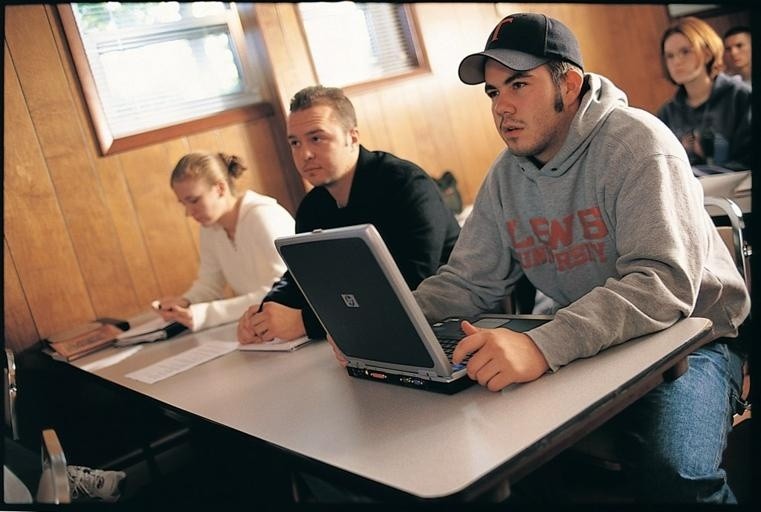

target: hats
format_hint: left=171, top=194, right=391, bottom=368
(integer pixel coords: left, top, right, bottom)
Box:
left=459, top=13, right=584, bottom=84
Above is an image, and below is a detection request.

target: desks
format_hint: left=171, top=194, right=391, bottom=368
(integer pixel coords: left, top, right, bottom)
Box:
left=42, top=317, right=716, bottom=512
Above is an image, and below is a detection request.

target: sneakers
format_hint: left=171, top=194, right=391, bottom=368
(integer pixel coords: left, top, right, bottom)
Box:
left=67, top=465, right=127, bottom=504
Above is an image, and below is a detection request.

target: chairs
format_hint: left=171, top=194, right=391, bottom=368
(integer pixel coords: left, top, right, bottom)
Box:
left=1, top=346, right=21, bottom=442
left=35, top=428, right=72, bottom=505
left=699, top=194, right=753, bottom=428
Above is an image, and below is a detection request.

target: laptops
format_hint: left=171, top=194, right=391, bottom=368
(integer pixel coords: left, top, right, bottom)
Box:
left=273, top=224, right=555, bottom=395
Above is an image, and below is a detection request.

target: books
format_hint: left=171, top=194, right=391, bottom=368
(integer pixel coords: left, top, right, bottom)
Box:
left=51, top=326, right=115, bottom=361
left=733, top=174, right=752, bottom=197
left=118, top=316, right=187, bottom=349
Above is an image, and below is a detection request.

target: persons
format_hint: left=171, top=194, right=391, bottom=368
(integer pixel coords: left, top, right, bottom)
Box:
left=237, top=85, right=463, bottom=347
left=328, top=12, right=754, bottom=506
left=2, top=436, right=127, bottom=501
left=150, top=153, right=296, bottom=333
left=654, top=15, right=752, bottom=177
left=722, top=26, right=751, bottom=91
